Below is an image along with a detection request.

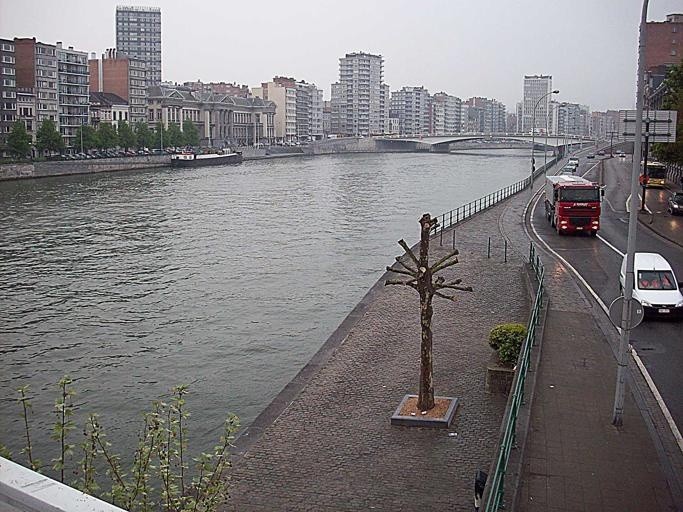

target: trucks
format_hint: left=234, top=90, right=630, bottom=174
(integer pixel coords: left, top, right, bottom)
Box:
left=616, top=253, right=683, bottom=321
left=543, top=176, right=606, bottom=238
left=326, top=135, right=338, bottom=140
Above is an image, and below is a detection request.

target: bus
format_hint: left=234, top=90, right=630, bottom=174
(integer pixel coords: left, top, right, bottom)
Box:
left=635, top=159, right=668, bottom=190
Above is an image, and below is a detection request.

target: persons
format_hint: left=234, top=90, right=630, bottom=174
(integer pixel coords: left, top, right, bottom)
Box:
left=663, top=273, right=671, bottom=286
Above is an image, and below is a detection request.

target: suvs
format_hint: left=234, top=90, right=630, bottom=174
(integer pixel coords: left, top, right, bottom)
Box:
left=561, top=156, right=579, bottom=175
left=666, top=191, right=682, bottom=215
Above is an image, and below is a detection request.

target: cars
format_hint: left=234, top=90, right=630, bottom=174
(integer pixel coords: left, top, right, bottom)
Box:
left=586, top=149, right=629, bottom=160
left=252, top=138, right=309, bottom=146
left=53, top=147, right=162, bottom=159
left=164, top=145, right=221, bottom=153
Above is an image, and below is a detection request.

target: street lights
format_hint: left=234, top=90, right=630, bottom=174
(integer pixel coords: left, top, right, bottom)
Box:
left=530, top=88, right=561, bottom=189
left=74, top=117, right=83, bottom=154
left=544, top=102, right=574, bottom=184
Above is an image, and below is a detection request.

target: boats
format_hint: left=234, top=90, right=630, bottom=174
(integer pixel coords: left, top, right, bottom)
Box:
left=166, top=147, right=242, bottom=168
